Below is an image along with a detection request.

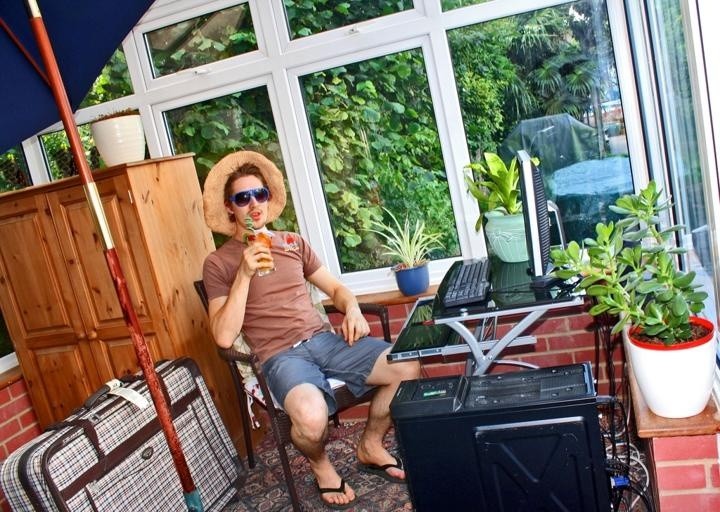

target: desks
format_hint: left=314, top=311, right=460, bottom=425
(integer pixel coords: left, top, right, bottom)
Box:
left=386, top=257, right=585, bottom=376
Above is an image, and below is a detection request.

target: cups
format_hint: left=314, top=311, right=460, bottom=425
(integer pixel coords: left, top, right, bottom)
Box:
left=245, top=230, right=276, bottom=278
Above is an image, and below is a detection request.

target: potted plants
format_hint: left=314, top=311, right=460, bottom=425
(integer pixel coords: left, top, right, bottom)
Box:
left=357, top=206, right=446, bottom=297
left=463, top=152, right=540, bottom=263
left=550, top=181, right=716, bottom=419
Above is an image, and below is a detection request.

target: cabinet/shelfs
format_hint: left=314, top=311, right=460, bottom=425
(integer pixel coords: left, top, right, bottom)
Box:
left=0, top=152, right=268, bottom=470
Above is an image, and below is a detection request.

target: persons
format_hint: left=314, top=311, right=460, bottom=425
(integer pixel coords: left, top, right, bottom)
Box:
left=202, top=151, right=420, bottom=506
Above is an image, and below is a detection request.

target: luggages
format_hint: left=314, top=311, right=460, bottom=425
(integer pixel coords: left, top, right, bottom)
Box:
left=0, top=356, right=250, bottom=512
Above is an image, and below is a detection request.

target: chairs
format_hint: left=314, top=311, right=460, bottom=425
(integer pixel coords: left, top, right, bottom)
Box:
left=194, top=280, right=391, bottom=512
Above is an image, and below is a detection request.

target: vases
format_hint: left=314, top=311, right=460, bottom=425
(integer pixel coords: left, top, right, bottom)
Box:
left=91, top=115, right=145, bottom=168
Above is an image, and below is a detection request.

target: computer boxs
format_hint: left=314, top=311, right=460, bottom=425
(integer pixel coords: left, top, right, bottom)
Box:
left=388, top=360, right=613, bottom=512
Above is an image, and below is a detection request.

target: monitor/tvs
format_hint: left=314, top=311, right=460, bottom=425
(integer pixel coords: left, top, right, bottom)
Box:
left=516, top=149, right=568, bottom=289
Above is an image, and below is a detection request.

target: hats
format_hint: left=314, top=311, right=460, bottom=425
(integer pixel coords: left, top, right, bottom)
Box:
left=202, top=150, right=288, bottom=237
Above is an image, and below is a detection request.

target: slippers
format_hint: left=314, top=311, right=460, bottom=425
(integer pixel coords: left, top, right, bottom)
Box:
left=357, top=453, right=408, bottom=484
left=314, top=477, right=360, bottom=510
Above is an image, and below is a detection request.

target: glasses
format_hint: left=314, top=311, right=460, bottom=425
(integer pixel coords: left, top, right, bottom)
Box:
left=229, top=186, right=270, bottom=208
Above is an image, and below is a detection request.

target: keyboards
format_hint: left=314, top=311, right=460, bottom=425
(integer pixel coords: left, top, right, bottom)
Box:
left=442, top=258, right=493, bottom=307
